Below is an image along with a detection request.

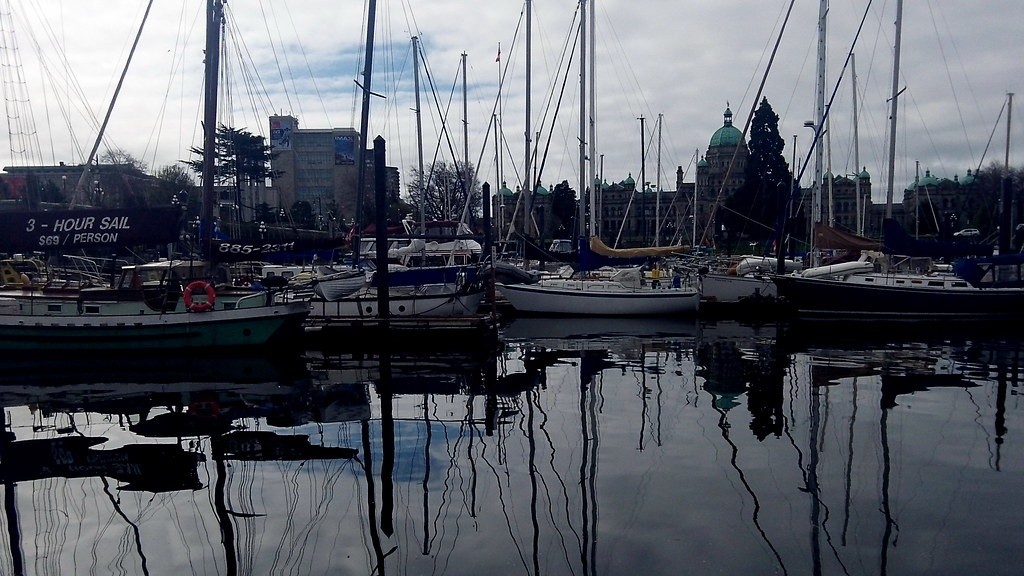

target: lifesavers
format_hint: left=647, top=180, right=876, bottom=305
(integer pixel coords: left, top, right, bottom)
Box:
left=189, top=401, right=219, bottom=421
left=183, top=280, right=216, bottom=311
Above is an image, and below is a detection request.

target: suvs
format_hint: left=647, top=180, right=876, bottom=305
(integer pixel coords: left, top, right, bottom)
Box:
left=952, top=229, right=979, bottom=237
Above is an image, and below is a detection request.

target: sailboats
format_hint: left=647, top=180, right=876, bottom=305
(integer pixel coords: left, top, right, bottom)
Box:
left=700, top=1, right=873, bottom=312
left=289, top=0, right=498, bottom=327
left=492, top=0, right=703, bottom=316
left=1, top=0, right=313, bottom=354
left=10, top=33, right=1002, bottom=275
left=765, top=0, right=1024, bottom=340
